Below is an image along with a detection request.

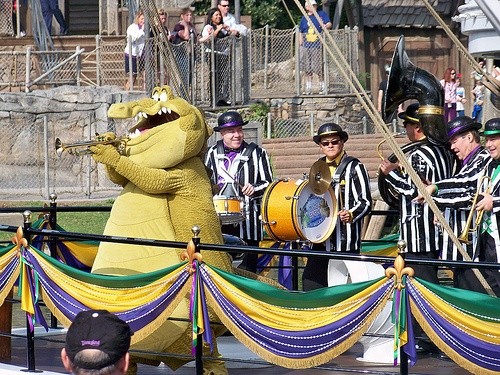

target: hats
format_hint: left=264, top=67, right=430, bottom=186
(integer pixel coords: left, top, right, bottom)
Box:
left=398, top=103, right=420, bottom=123
left=313, top=123, right=348, bottom=144
left=213, top=112, right=249, bottom=132
left=479, top=118, right=500, bottom=136
left=305, top=0, right=317, bottom=7
left=447, top=116, right=482, bottom=140
left=385, top=68, right=390, bottom=74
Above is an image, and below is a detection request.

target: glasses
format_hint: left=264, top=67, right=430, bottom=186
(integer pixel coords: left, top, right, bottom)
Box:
left=222, top=5, right=230, bottom=7
left=403, top=121, right=415, bottom=128
left=159, top=12, right=167, bottom=15
left=321, top=139, right=341, bottom=146
left=451, top=73, right=455, bottom=75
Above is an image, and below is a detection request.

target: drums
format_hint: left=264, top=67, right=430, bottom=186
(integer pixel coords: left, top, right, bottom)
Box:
left=261, top=178, right=338, bottom=244
left=211, top=195, right=246, bottom=224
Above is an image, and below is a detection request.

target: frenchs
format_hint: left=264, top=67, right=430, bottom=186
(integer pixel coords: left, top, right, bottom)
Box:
left=375, top=34, right=445, bottom=209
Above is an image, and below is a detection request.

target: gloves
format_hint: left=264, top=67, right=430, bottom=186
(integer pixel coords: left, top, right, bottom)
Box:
left=65, top=310, right=134, bottom=369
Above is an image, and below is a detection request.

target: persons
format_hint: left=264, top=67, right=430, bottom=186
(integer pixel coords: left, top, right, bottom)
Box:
left=411, top=116, right=494, bottom=361
left=380, top=103, right=461, bottom=352
left=150, top=8, right=176, bottom=89
left=302, top=122, right=372, bottom=292
left=3, top=0, right=26, bottom=38
left=204, top=111, right=274, bottom=273
left=473, top=118, right=500, bottom=273
left=203, top=0, right=247, bottom=107
left=169, top=7, right=212, bottom=101
left=60, top=310, right=134, bottom=375
left=299, top=0, right=332, bottom=95
left=440, top=61, right=500, bottom=122
left=124, top=9, right=147, bottom=91
left=40, top=0, right=69, bottom=35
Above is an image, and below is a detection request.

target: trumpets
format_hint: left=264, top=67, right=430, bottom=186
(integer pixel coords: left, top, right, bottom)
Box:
left=54, top=137, right=131, bottom=161
left=457, top=166, right=492, bottom=243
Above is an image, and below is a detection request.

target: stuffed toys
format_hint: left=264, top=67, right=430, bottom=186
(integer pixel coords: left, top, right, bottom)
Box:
left=87, top=85, right=290, bottom=375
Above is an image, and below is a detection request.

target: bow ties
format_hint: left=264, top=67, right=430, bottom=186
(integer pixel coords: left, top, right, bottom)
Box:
left=224, top=147, right=240, bottom=153
left=459, top=159, right=463, bottom=167
left=327, top=160, right=337, bottom=167
left=493, top=157, right=500, bottom=169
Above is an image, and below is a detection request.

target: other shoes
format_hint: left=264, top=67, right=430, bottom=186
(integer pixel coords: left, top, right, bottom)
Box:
left=318, top=85, right=330, bottom=94
left=227, top=100, right=241, bottom=105
left=414, top=339, right=432, bottom=352
left=303, top=86, right=314, bottom=95
left=437, top=352, right=452, bottom=362
left=218, top=99, right=230, bottom=106
